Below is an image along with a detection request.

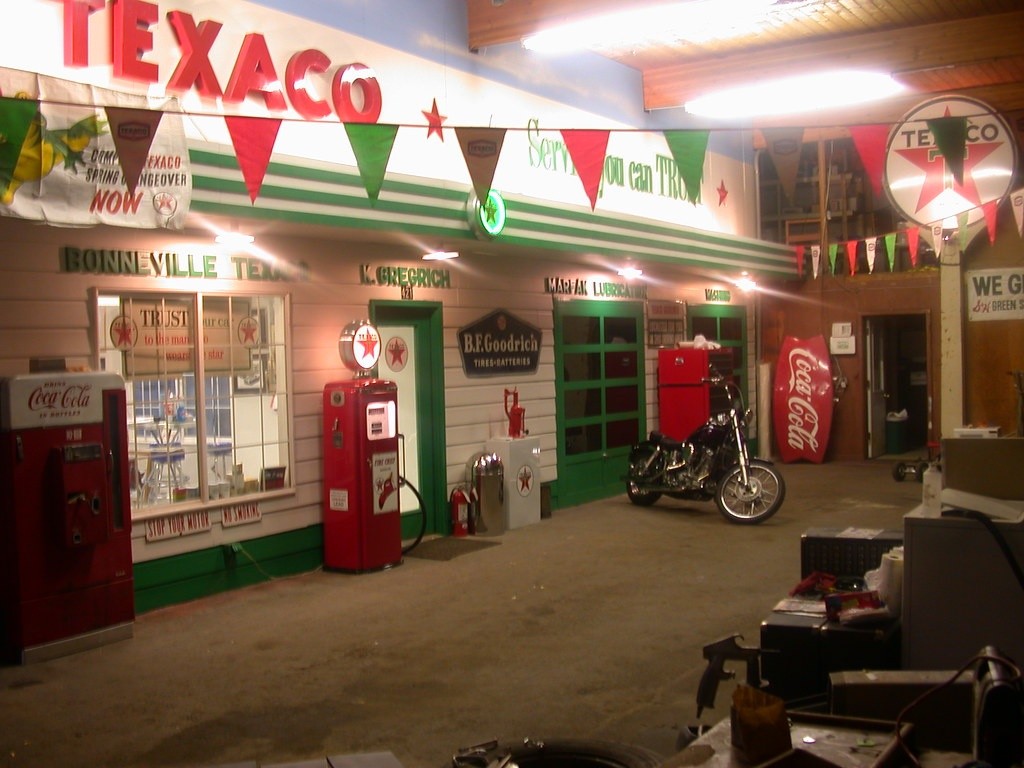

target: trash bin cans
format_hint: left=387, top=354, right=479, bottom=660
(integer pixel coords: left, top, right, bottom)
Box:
left=465, top=451, right=505, bottom=537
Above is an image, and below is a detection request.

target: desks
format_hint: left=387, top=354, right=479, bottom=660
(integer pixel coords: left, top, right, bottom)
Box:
left=129, top=419, right=196, bottom=505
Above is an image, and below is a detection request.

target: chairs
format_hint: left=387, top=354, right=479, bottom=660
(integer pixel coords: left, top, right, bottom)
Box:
left=786, top=219, right=822, bottom=276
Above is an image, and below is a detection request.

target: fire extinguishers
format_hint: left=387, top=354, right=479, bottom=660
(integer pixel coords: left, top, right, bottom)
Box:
left=447, top=483, right=469, bottom=538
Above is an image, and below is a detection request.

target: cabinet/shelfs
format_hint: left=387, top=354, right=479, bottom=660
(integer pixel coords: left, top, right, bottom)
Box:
left=759, top=171, right=853, bottom=273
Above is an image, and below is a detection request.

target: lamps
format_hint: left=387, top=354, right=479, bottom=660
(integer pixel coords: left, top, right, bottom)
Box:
left=422, top=250, right=460, bottom=260
left=618, top=268, right=643, bottom=277
left=215, top=233, right=255, bottom=244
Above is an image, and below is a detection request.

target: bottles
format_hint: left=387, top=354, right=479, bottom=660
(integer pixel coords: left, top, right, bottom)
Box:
left=234, top=464, right=245, bottom=494
left=922, top=462, right=943, bottom=519
left=177, top=396, right=185, bottom=423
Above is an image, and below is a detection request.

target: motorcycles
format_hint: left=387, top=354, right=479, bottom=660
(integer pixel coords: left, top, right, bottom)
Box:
left=621, top=364, right=785, bottom=524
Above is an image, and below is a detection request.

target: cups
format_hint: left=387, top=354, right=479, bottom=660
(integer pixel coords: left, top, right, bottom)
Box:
left=165, top=415, right=173, bottom=423
left=218, top=482, right=231, bottom=499
left=209, top=484, right=220, bottom=500
left=186, top=415, right=193, bottom=423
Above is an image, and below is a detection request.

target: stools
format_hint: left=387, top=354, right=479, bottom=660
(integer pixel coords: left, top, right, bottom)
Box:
left=145, top=441, right=232, bottom=505
